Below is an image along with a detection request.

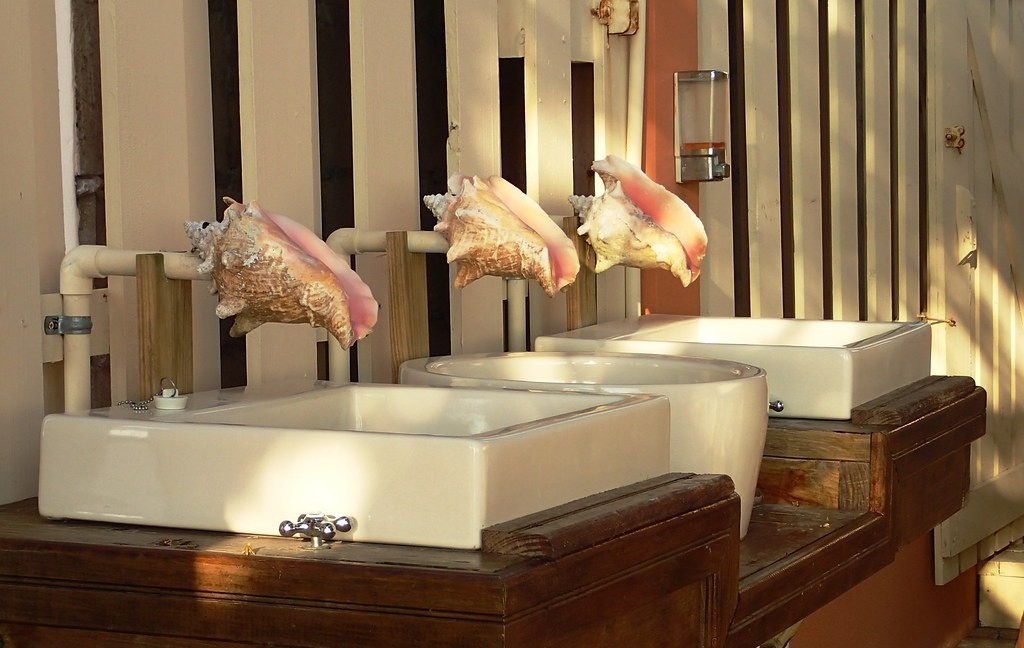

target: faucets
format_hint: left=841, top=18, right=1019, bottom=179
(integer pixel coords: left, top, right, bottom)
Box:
left=503, top=153, right=712, bottom=353
left=321, top=172, right=583, bottom=382
left=56, top=194, right=381, bottom=415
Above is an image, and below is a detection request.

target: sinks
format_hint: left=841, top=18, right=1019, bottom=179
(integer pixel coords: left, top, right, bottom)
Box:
left=35, top=379, right=673, bottom=553
left=535, top=312, right=933, bottom=422
left=395, top=350, right=771, bottom=540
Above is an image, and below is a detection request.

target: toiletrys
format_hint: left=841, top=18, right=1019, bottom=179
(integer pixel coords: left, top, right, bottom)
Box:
left=673, top=69, right=732, bottom=185
